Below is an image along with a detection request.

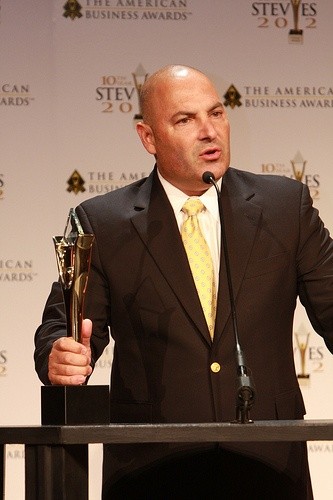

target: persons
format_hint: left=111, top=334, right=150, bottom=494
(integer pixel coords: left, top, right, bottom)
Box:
left=33, top=62, right=332, bottom=500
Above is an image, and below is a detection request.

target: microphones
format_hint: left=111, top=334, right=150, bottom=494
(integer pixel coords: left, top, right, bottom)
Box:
left=202, top=170, right=256, bottom=424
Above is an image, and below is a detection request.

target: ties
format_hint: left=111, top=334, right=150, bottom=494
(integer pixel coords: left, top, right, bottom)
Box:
left=178, top=197, right=217, bottom=344
